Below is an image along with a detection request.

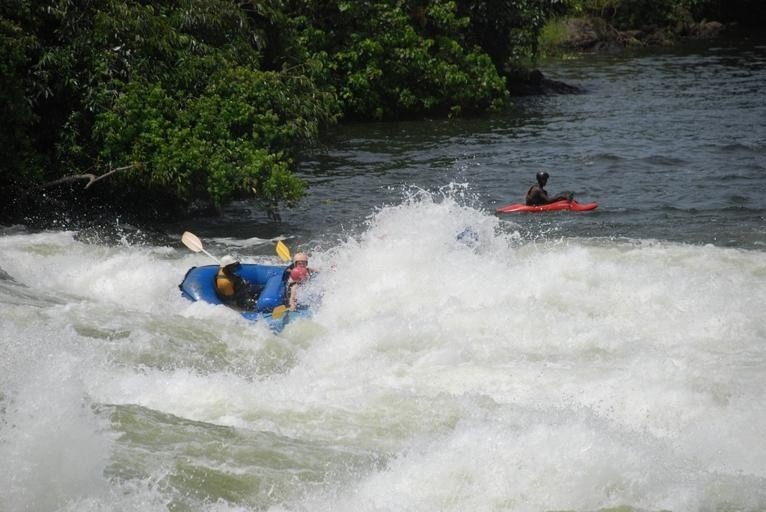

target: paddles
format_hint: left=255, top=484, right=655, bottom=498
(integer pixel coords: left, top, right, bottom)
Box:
left=275, top=240, right=293, bottom=262
left=181, top=231, right=220, bottom=265
left=272, top=304, right=290, bottom=319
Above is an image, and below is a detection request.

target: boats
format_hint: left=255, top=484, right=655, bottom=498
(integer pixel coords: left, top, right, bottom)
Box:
left=495, top=199, right=598, bottom=214
left=178, top=264, right=321, bottom=322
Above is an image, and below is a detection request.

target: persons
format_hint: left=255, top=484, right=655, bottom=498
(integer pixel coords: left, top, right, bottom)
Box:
left=284, top=251, right=322, bottom=312
left=526, top=171, right=569, bottom=205
left=217, top=255, right=253, bottom=308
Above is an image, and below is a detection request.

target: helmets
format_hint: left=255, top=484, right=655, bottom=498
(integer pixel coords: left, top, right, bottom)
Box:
left=219, top=255, right=238, bottom=268
left=293, top=253, right=309, bottom=266
left=535, top=171, right=549, bottom=180
left=290, top=266, right=308, bottom=283
